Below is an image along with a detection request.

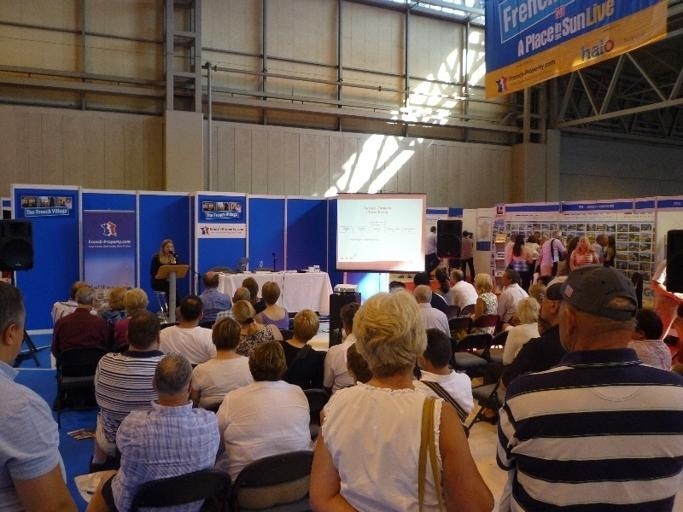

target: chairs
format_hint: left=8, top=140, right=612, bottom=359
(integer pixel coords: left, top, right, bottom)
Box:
left=40, top=287, right=682, bottom=512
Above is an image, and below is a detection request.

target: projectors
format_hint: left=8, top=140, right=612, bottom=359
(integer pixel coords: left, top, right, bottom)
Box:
left=334, top=284, right=358, bottom=292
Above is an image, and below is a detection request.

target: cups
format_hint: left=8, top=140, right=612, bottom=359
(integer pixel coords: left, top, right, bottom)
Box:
left=308, top=266, right=314, bottom=273
left=312, top=265, right=320, bottom=273
left=258, top=260, right=263, bottom=269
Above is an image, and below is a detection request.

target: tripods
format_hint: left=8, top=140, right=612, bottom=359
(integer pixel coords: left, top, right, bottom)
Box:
left=10, top=272, right=40, bottom=368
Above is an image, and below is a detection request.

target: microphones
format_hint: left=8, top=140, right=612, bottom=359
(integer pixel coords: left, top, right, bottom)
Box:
left=271, top=252, right=278, bottom=272
left=169, top=250, right=179, bottom=258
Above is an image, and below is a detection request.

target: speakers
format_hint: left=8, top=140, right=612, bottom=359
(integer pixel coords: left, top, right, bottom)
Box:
left=437, top=220, right=462, bottom=257
left=0, top=220, right=34, bottom=271
left=667, top=230, right=683, bottom=293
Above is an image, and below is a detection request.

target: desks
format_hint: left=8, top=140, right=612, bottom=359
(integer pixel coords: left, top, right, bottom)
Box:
left=211, top=266, right=335, bottom=317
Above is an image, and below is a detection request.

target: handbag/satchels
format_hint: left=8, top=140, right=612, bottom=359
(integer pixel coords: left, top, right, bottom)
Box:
left=550, top=260, right=560, bottom=276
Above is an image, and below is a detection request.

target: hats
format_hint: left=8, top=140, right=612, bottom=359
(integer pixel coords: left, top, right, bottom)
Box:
left=544, top=260, right=641, bottom=323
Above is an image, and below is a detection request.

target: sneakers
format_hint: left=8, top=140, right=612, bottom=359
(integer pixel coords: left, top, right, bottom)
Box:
left=477, top=410, right=500, bottom=426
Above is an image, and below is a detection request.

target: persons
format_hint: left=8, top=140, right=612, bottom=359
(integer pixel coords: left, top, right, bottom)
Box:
left=0, top=281, right=79, bottom=512
left=436, top=266, right=682, bottom=511
left=151, top=239, right=182, bottom=313
left=51, top=272, right=494, bottom=511
left=425, top=227, right=441, bottom=277
left=504, top=228, right=616, bottom=292
left=459, top=231, right=475, bottom=283
